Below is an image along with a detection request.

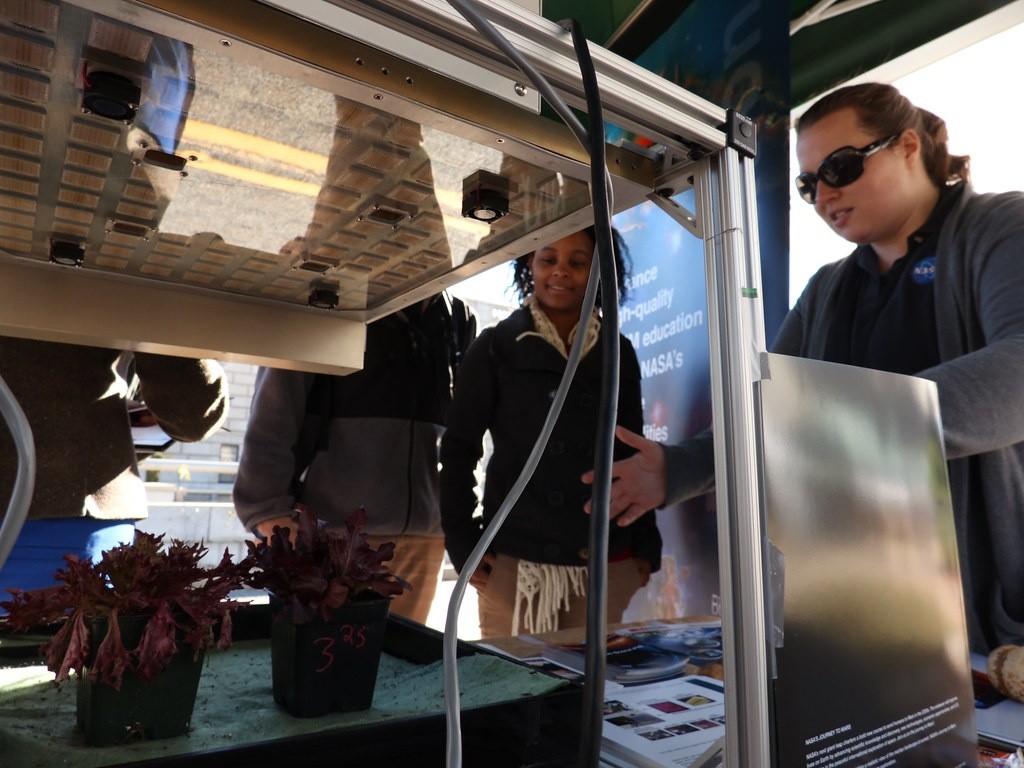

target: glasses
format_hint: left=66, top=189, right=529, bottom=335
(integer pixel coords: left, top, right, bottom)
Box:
left=795, top=131, right=903, bottom=204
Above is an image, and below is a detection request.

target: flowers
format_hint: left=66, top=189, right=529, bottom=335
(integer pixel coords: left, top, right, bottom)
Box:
left=11, top=528, right=252, bottom=691
left=238, top=513, right=410, bottom=607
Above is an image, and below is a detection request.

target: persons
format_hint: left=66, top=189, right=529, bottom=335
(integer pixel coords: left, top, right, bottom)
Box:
left=0, top=336, right=229, bottom=616
left=438, top=223, right=663, bottom=640
left=583, top=80, right=1023, bottom=658
left=233, top=289, right=484, bottom=626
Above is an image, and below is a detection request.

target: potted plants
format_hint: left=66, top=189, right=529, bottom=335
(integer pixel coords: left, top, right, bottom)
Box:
left=63, top=608, right=215, bottom=744
left=268, top=582, right=380, bottom=719
